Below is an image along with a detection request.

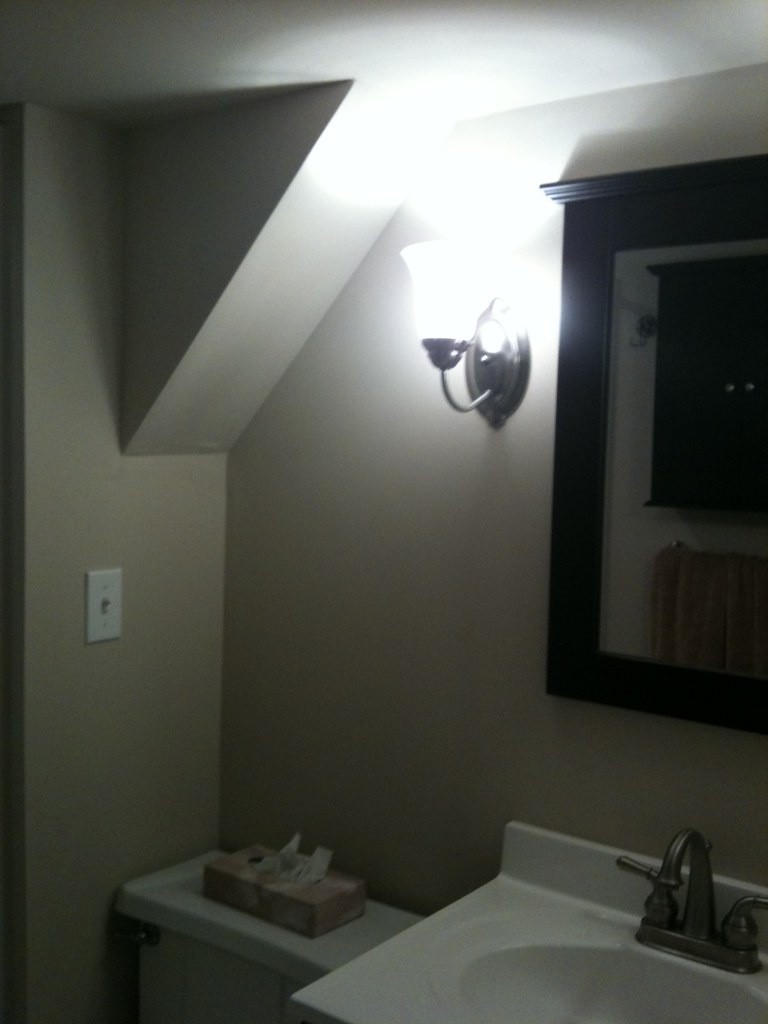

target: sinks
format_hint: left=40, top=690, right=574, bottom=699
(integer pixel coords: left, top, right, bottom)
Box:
left=289, top=812, right=766, bottom=1024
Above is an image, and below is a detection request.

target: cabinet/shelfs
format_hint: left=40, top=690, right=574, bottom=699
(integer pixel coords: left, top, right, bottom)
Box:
left=644, top=250, right=768, bottom=513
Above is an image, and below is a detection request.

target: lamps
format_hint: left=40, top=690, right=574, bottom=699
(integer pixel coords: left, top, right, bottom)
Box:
left=401, top=240, right=531, bottom=433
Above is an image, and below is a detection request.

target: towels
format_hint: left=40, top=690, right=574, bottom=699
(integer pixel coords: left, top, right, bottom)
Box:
left=650, top=543, right=768, bottom=678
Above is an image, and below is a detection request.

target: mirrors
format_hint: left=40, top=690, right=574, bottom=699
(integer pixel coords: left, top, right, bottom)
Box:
left=540, top=150, right=768, bottom=734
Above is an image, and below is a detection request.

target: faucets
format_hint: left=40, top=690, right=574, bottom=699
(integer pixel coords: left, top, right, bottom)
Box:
left=654, top=825, right=722, bottom=963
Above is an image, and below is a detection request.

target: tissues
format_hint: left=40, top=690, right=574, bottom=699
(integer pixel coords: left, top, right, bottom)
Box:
left=201, top=829, right=371, bottom=942
